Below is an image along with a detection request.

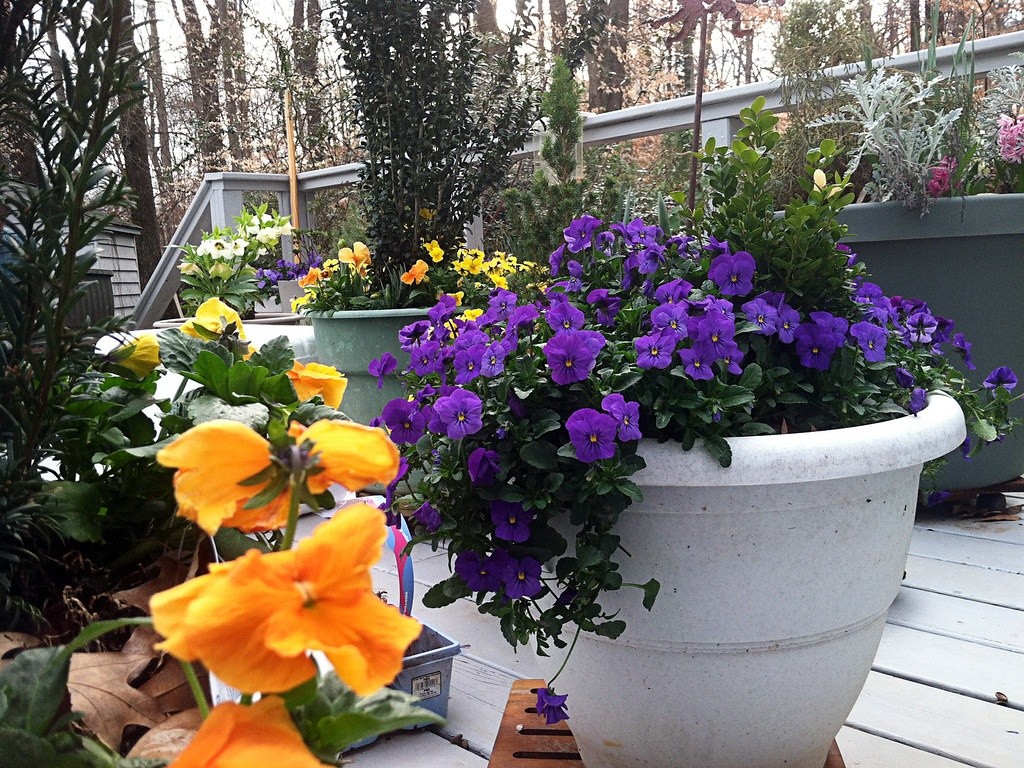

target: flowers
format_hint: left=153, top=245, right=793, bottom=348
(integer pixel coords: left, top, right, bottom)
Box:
left=54, top=70, right=1024, bottom=768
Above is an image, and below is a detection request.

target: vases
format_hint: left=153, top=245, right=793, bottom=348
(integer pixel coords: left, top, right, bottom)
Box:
left=514, top=390, right=968, bottom=768
left=336, top=603, right=460, bottom=753
left=154, top=312, right=306, bottom=329
left=278, top=281, right=306, bottom=312
left=771, top=194, right=1024, bottom=488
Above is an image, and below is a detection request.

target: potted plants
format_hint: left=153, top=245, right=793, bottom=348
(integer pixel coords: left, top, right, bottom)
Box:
left=304, top=1, right=607, bottom=497
